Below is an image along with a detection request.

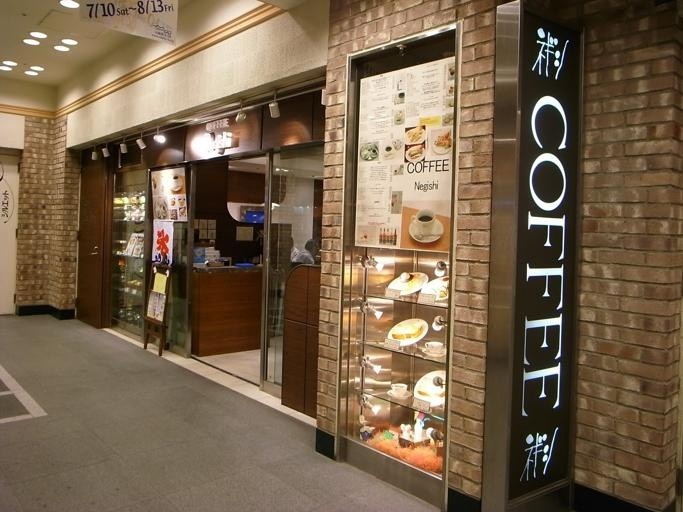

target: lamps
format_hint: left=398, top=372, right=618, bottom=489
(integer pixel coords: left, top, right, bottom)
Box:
left=424, top=260, right=448, bottom=446
left=234, top=88, right=281, bottom=125
left=357, top=252, right=384, bottom=418
left=90, top=127, right=166, bottom=162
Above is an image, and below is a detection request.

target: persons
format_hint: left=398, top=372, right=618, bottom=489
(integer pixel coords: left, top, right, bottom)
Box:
left=290, top=235, right=320, bottom=264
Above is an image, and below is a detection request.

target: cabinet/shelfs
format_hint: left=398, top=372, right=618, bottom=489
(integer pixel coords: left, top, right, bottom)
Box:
left=110, top=217, right=143, bottom=332
left=357, top=247, right=447, bottom=479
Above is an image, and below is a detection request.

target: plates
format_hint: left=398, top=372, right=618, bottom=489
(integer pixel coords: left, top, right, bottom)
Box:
left=404, top=128, right=453, bottom=163
left=422, top=349, right=447, bottom=357
left=387, top=390, right=413, bottom=400
left=407, top=218, right=445, bottom=243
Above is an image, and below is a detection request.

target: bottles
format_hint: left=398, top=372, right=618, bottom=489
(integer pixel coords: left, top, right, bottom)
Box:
left=378, top=228, right=397, bottom=246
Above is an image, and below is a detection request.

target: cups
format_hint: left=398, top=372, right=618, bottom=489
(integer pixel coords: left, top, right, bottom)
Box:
left=411, top=207, right=436, bottom=236
left=425, top=341, right=443, bottom=354
left=392, top=383, right=407, bottom=396
